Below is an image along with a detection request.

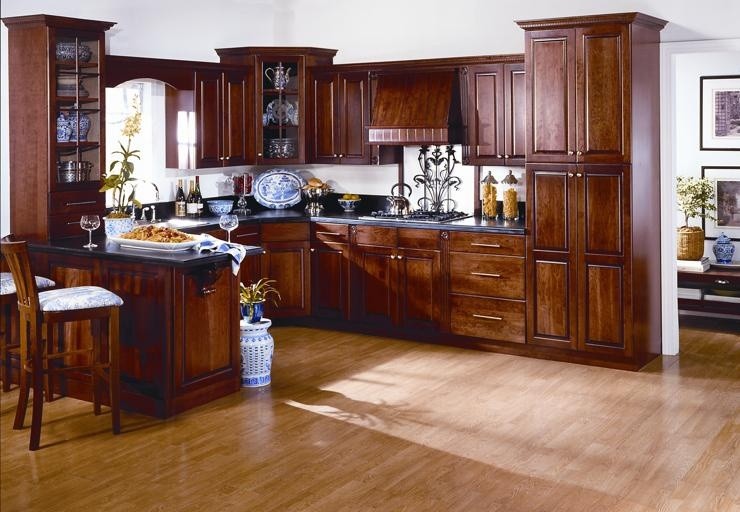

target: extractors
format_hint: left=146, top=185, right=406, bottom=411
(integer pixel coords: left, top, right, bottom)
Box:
left=362, top=69, right=460, bottom=146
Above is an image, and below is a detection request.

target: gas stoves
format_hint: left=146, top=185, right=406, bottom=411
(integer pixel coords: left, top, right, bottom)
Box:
left=356, top=207, right=473, bottom=225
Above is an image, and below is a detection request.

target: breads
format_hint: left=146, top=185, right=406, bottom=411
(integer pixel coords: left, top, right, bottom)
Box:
left=303, top=177, right=328, bottom=190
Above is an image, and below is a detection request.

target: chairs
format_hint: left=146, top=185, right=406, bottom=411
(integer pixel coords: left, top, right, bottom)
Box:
left=0, top=234, right=125, bottom=451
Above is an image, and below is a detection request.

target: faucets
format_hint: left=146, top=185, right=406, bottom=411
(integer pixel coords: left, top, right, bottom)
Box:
left=131, top=179, right=161, bottom=222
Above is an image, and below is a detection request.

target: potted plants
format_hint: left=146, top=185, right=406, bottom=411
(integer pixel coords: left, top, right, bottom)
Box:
left=234, top=276, right=279, bottom=322
left=674, top=177, right=718, bottom=261
left=100, top=92, right=142, bottom=237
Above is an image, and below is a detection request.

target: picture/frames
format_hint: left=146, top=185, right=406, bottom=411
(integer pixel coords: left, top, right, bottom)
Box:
left=700, top=75, right=740, bottom=151
left=701, top=166, right=740, bottom=241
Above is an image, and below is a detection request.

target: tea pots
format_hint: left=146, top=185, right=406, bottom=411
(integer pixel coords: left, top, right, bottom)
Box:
left=265, top=61, right=291, bottom=91
left=384, top=182, right=412, bottom=214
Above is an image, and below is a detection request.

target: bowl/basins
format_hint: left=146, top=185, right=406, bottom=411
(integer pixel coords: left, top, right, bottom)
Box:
left=296, top=186, right=335, bottom=216
left=55, top=44, right=91, bottom=63
left=206, top=200, right=234, bottom=216
left=339, top=199, right=361, bottom=212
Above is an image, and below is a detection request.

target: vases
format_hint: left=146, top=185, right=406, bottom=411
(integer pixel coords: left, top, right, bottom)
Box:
left=241, top=319, right=274, bottom=389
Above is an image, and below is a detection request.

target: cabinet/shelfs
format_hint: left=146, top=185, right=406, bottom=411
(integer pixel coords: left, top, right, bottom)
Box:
left=194, top=47, right=374, bottom=167
left=19, top=241, right=263, bottom=420
left=188, top=222, right=525, bottom=355
left=460, top=57, right=526, bottom=167
left=524, top=12, right=669, bottom=371
left=677, top=271, right=740, bottom=330
left=1, top=14, right=117, bottom=237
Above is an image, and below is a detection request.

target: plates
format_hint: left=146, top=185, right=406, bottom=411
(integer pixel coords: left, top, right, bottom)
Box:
left=56, top=74, right=90, bottom=99
left=108, top=237, right=201, bottom=252
left=266, top=98, right=293, bottom=125
left=252, top=167, right=308, bottom=209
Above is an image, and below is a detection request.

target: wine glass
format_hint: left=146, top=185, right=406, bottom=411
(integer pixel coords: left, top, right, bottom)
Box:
left=218, top=214, right=240, bottom=242
left=81, top=214, right=102, bottom=248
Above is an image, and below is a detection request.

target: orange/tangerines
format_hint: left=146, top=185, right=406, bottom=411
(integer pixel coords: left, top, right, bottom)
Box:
left=342, top=194, right=360, bottom=200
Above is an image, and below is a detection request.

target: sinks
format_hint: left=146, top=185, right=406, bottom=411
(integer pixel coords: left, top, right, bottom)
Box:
left=137, top=217, right=210, bottom=230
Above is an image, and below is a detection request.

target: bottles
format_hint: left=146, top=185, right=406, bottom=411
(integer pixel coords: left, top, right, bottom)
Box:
left=185, top=180, right=199, bottom=219
left=174, top=178, right=186, bottom=217
left=193, top=175, right=203, bottom=207
left=479, top=171, right=500, bottom=220
left=501, top=170, right=519, bottom=222
left=67, top=106, right=88, bottom=142
left=712, top=233, right=735, bottom=265
left=56, top=113, right=72, bottom=143
